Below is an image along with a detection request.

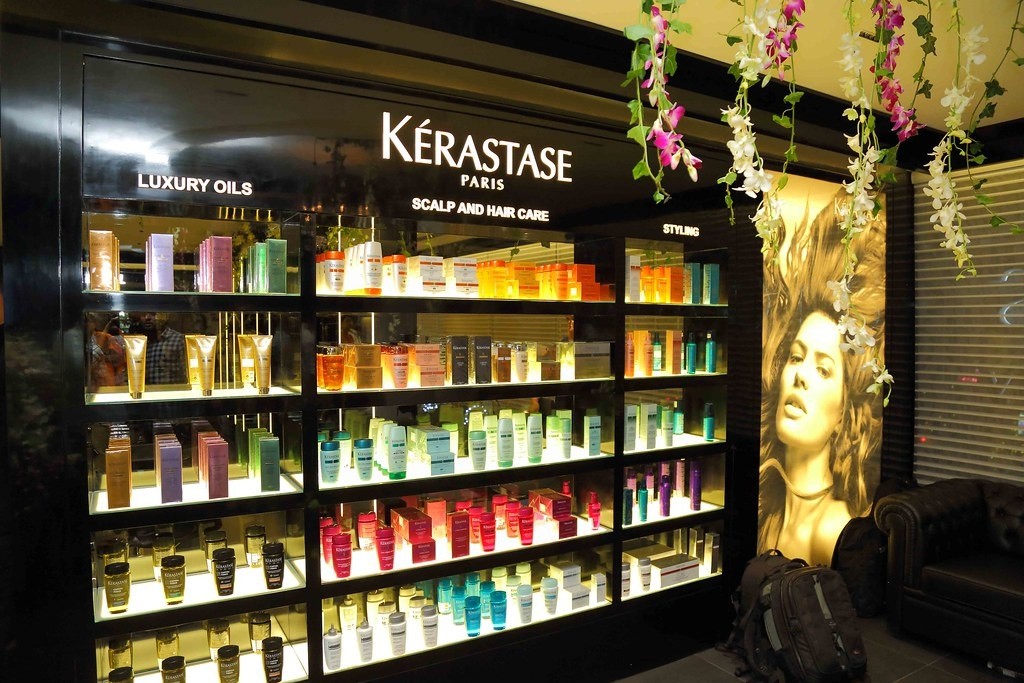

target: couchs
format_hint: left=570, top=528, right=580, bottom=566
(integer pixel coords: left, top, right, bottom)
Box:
left=874, top=479, right=1024, bottom=671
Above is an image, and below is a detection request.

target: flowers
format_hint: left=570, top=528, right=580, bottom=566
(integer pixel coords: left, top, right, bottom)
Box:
left=622, top=0, right=1024, bottom=281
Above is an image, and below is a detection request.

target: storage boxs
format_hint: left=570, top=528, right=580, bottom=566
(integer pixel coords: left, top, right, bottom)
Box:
left=667, top=263, right=719, bottom=305
left=407, top=255, right=479, bottom=298
left=674, top=528, right=720, bottom=575
left=665, top=330, right=682, bottom=375
left=154, top=434, right=182, bottom=505
left=378, top=500, right=407, bottom=527
left=625, top=402, right=656, bottom=452
left=429, top=334, right=491, bottom=385
left=622, top=544, right=699, bottom=589
left=246, top=240, right=287, bottom=293
left=410, top=344, right=445, bottom=387
left=105, top=438, right=131, bottom=509
left=634, top=331, right=651, bottom=377
left=199, top=236, right=233, bottom=292
left=146, top=234, right=174, bottom=292
left=584, top=416, right=601, bottom=457
left=557, top=409, right=572, bottom=447
left=448, top=510, right=469, bottom=559
left=625, top=255, right=640, bottom=302
left=89, top=229, right=121, bottom=292
left=340, top=344, right=383, bottom=390
left=549, top=562, right=606, bottom=611
left=198, top=432, right=229, bottom=499
left=248, top=428, right=280, bottom=493
left=424, top=499, right=446, bottom=529
left=528, top=488, right=578, bottom=538
left=407, top=426, right=455, bottom=476
left=566, top=264, right=600, bottom=302
left=390, top=507, right=436, bottom=566
left=505, top=261, right=539, bottom=299
left=528, top=341, right=611, bottom=382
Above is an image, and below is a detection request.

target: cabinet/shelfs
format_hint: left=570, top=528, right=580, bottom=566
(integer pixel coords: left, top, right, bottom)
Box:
left=80, top=197, right=763, bottom=683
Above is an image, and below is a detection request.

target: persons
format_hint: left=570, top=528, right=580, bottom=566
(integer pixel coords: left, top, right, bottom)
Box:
left=140, top=313, right=187, bottom=384
left=64, top=313, right=125, bottom=387
left=493, top=397, right=540, bottom=416
left=758, top=189, right=885, bottom=566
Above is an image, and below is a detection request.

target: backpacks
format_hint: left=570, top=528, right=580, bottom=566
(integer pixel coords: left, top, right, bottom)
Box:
left=714, top=549, right=867, bottom=683
left=831, top=515, right=892, bottom=617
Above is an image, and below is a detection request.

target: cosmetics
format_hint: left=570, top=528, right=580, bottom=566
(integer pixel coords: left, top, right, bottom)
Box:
left=323, top=560, right=607, bottom=671
left=623, top=401, right=718, bottom=451
left=316, top=242, right=616, bottom=301
left=107, top=612, right=282, bottom=683
left=318, top=335, right=610, bottom=390
left=321, top=477, right=602, bottom=578
left=622, top=458, right=703, bottom=525
left=624, top=255, right=720, bottom=305
left=90, top=231, right=286, bottom=294
left=621, top=527, right=719, bottom=597
left=124, top=334, right=273, bottom=399
left=107, top=427, right=280, bottom=508
left=625, top=330, right=717, bottom=377
left=317, top=407, right=601, bottom=484
left=100, top=528, right=286, bottom=613
